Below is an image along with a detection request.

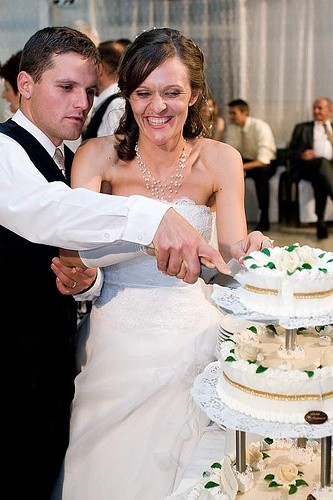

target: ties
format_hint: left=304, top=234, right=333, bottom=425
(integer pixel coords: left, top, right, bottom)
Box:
left=323, top=122, right=333, bottom=146
left=241, top=131, right=246, bottom=157
left=53, top=148, right=67, bottom=180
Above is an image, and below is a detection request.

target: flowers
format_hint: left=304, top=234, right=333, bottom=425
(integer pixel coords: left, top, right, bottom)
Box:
left=244, top=446, right=310, bottom=495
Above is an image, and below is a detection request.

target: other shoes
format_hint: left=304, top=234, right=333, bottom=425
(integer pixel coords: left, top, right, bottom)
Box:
left=255, top=223, right=270, bottom=231
left=317, top=226, right=328, bottom=238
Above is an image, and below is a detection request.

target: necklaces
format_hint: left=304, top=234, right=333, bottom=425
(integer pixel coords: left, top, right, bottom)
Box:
left=134, top=138, right=187, bottom=200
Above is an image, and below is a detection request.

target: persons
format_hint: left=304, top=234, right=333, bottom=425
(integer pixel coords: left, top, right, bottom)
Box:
left=0, top=27, right=231, bottom=500
left=0, top=50, right=23, bottom=114
left=60, top=26, right=275, bottom=500
left=289, top=97, right=333, bottom=239
left=224, top=100, right=279, bottom=232
left=81, top=38, right=132, bottom=140
left=200, top=96, right=226, bottom=141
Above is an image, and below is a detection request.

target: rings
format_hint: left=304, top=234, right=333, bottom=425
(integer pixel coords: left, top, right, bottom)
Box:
left=71, top=282, right=76, bottom=289
left=263, top=239, right=274, bottom=246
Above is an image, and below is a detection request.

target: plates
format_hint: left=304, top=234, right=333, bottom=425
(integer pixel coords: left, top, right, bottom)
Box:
left=214, top=316, right=267, bottom=361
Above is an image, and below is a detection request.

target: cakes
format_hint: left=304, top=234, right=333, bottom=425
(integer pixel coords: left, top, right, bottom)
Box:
left=188, top=243, right=333, bottom=500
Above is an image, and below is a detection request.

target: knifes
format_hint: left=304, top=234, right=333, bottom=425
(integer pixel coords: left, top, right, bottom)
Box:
left=198, top=265, right=242, bottom=289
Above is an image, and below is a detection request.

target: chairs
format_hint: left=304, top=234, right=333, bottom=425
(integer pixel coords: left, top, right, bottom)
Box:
left=243, top=142, right=333, bottom=228
